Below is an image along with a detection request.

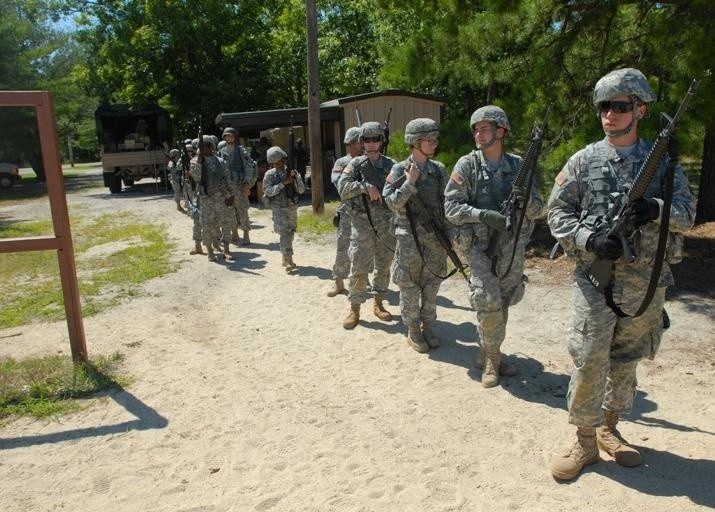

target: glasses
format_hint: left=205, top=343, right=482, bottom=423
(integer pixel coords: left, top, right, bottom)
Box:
left=599, top=101, right=633, bottom=114
left=421, top=139, right=439, bottom=146
left=363, top=137, right=381, bottom=143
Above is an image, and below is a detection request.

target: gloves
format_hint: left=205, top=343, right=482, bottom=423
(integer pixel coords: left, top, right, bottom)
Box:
left=586, top=228, right=624, bottom=262
left=479, top=209, right=506, bottom=234
left=512, top=186, right=528, bottom=200
left=624, top=197, right=660, bottom=230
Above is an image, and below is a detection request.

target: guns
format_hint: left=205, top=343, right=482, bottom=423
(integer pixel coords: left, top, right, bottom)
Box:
left=486, top=102, right=556, bottom=277
left=355, top=161, right=388, bottom=209
left=163, top=153, right=176, bottom=164
left=179, top=133, right=195, bottom=191
left=386, top=173, right=468, bottom=281
left=198, top=114, right=208, bottom=195
left=381, top=108, right=392, bottom=156
left=285, top=114, right=296, bottom=197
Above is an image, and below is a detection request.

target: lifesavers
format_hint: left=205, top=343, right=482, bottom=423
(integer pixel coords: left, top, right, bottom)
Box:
left=587, top=79, right=701, bottom=295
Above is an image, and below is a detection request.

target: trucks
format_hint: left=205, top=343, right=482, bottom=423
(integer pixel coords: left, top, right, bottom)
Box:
left=95, top=103, right=178, bottom=194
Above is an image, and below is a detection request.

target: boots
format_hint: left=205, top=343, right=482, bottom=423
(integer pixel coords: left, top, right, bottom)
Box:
left=240, top=230, right=250, bottom=246
left=223, top=243, right=236, bottom=260
left=423, top=322, right=440, bottom=349
left=476, top=345, right=518, bottom=376
left=231, top=229, right=239, bottom=242
left=596, top=408, right=642, bottom=467
left=407, top=325, right=430, bottom=353
left=282, top=255, right=296, bottom=267
left=285, top=256, right=296, bottom=272
left=212, top=242, right=223, bottom=252
left=551, top=426, right=600, bottom=480
left=482, top=345, right=501, bottom=388
left=342, top=302, right=361, bottom=330
left=373, top=293, right=392, bottom=321
left=177, top=201, right=184, bottom=211
left=189, top=240, right=203, bottom=255
left=206, top=244, right=215, bottom=262
left=327, top=279, right=344, bottom=297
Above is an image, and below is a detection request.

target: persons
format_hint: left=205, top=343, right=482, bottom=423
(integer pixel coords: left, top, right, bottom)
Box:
left=383, top=118, right=460, bottom=352
left=263, top=146, right=305, bottom=271
left=251, top=138, right=271, bottom=201
left=327, top=126, right=373, bottom=297
left=296, top=139, right=310, bottom=186
left=167, top=125, right=256, bottom=261
left=339, top=120, right=397, bottom=329
left=443, top=106, right=545, bottom=389
left=547, top=68, right=697, bottom=479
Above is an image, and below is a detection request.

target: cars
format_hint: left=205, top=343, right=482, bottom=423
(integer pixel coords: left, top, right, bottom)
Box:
left=0, top=156, right=19, bottom=190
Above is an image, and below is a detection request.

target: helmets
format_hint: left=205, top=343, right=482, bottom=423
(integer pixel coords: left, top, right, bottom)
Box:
left=184, top=139, right=192, bottom=145
left=266, top=145, right=288, bottom=164
left=222, top=127, right=239, bottom=139
left=469, top=105, right=511, bottom=132
left=192, top=138, right=199, bottom=150
left=358, top=121, right=386, bottom=144
left=343, top=127, right=362, bottom=145
left=217, top=140, right=227, bottom=150
left=210, top=135, right=218, bottom=146
left=169, top=149, right=181, bottom=158
left=592, top=68, right=657, bottom=116
left=404, top=117, right=439, bottom=144
left=197, top=135, right=216, bottom=150
